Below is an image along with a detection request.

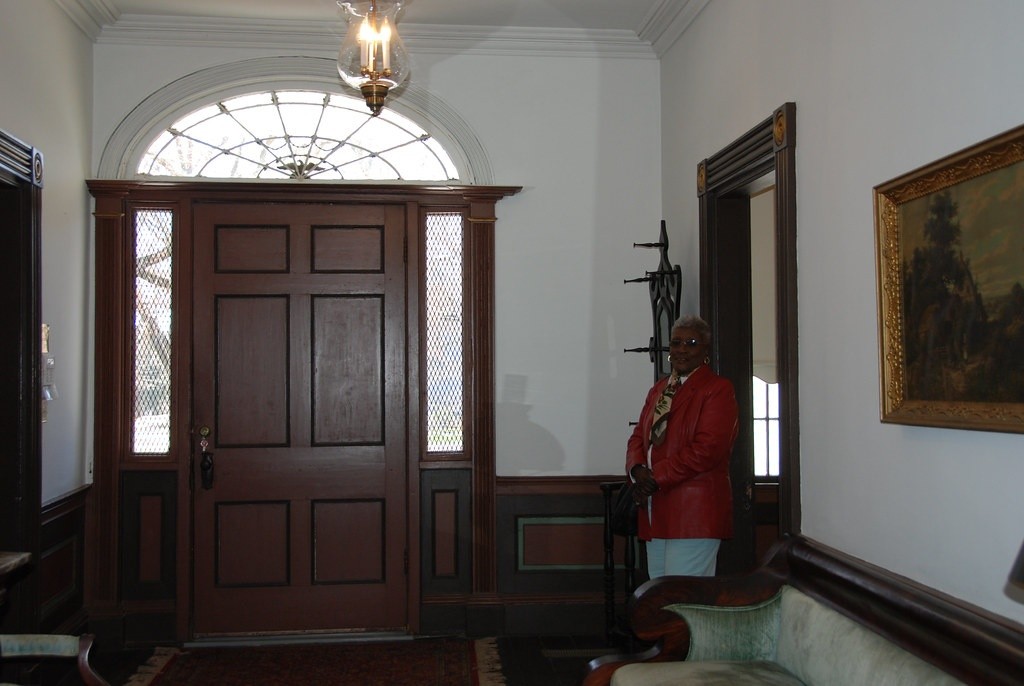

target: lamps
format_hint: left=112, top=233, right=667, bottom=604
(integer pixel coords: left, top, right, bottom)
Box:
left=333, top=1, right=412, bottom=118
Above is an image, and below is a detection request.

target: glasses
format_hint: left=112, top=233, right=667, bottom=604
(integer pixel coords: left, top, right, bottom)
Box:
left=669, top=338, right=704, bottom=349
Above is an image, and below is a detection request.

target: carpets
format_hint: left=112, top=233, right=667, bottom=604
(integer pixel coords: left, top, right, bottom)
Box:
left=122, top=632, right=510, bottom=686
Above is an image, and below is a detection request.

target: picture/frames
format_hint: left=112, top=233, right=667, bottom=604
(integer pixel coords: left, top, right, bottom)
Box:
left=870, top=122, right=1024, bottom=434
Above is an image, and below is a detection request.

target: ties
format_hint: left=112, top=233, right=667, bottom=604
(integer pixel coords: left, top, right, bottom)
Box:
left=651, top=371, right=681, bottom=444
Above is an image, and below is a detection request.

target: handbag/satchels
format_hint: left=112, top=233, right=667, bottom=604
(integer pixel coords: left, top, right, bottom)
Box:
left=612, top=474, right=640, bottom=536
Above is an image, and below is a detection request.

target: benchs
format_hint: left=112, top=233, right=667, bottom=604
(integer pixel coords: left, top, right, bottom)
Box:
left=573, top=525, right=1023, bottom=686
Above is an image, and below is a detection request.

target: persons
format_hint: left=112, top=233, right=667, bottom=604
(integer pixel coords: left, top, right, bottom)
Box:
left=622, top=316, right=737, bottom=575
